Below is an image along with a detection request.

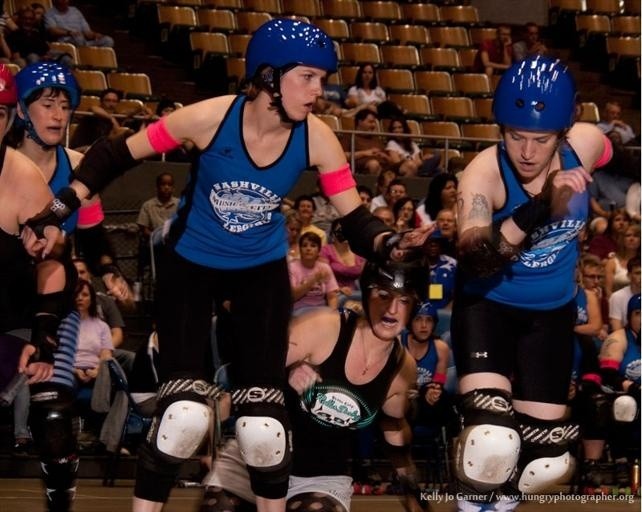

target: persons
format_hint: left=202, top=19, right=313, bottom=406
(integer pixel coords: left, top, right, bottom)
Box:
left=1, top=2, right=641, bottom=512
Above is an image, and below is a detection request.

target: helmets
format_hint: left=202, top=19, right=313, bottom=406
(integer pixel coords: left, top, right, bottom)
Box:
left=416, top=301, right=439, bottom=322
left=0, top=60, right=18, bottom=105
left=627, top=293, right=640, bottom=314
left=15, top=60, right=81, bottom=126
left=492, top=54, right=576, bottom=131
left=245, top=18, right=338, bottom=84
left=360, top=256, right=429, bottom=306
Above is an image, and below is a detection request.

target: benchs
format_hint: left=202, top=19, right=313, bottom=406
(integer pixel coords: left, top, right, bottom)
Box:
left=0, top=0, right=641, bottom=496
